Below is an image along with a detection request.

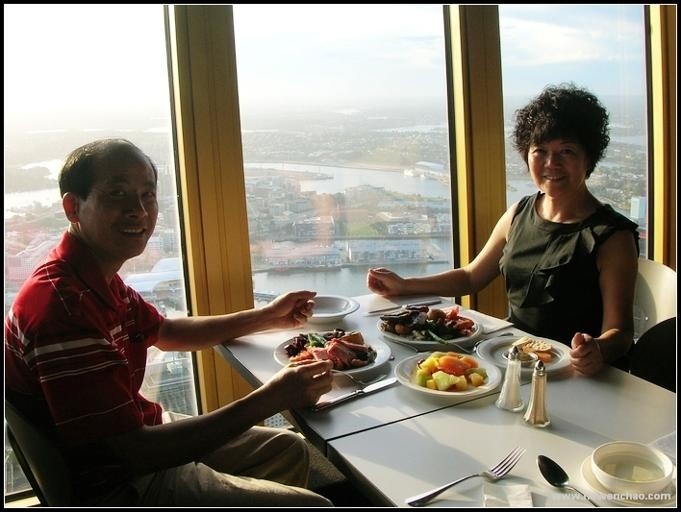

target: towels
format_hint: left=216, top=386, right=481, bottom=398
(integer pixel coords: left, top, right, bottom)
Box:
left=649, top=431, right=675, bottom=464
left=480, top=480, right=534, bottom=508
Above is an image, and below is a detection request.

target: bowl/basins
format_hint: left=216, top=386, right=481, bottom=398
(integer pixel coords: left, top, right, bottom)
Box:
left=592, top=440, right=674, bottom=496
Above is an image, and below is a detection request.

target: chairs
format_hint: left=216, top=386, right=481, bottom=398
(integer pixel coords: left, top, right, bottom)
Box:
left=635, top=257, right=678, bottom=341
left=8, top=375, right=112, bottom=506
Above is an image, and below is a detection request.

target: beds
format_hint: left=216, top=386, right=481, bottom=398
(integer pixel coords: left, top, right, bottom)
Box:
left=213, top=288, right=587, bottom=449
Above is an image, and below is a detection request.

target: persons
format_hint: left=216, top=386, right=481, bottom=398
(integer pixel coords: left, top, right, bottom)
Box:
left=3, top=137, right=334, bottom=506
left=366, top=83, right=637, bottom=377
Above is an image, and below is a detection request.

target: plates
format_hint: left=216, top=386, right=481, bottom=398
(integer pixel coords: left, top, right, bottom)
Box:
left=274, top=330, right=391, bottom=375
left=474, top=335, right=573, bottom=373
left=307, top=295, right=360, bottom=323
left=394, top=352, right=503, bottom=396
left=582, top=457, right=677, bottom=507
left=378, top=314, right=482, bottom=345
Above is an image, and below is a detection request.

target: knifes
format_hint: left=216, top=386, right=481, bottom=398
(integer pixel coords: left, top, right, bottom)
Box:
left=369, top=300, right=442, bottom=314
left=312, top=376, right=399, bottom=412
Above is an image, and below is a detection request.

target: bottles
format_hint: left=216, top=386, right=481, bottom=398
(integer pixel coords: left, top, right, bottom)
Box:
left=495, top=346, right=525, bottom=412
left=524, top=359, right=552, bottom=428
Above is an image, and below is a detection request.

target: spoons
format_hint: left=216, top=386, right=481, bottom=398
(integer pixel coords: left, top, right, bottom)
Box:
left=537, top=454, right=602, bottom=507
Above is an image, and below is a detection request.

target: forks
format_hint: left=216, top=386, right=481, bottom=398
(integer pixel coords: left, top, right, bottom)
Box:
left=313, top=370, right=387, bottom=385
left=406, top=446, right=527, bottom=506
